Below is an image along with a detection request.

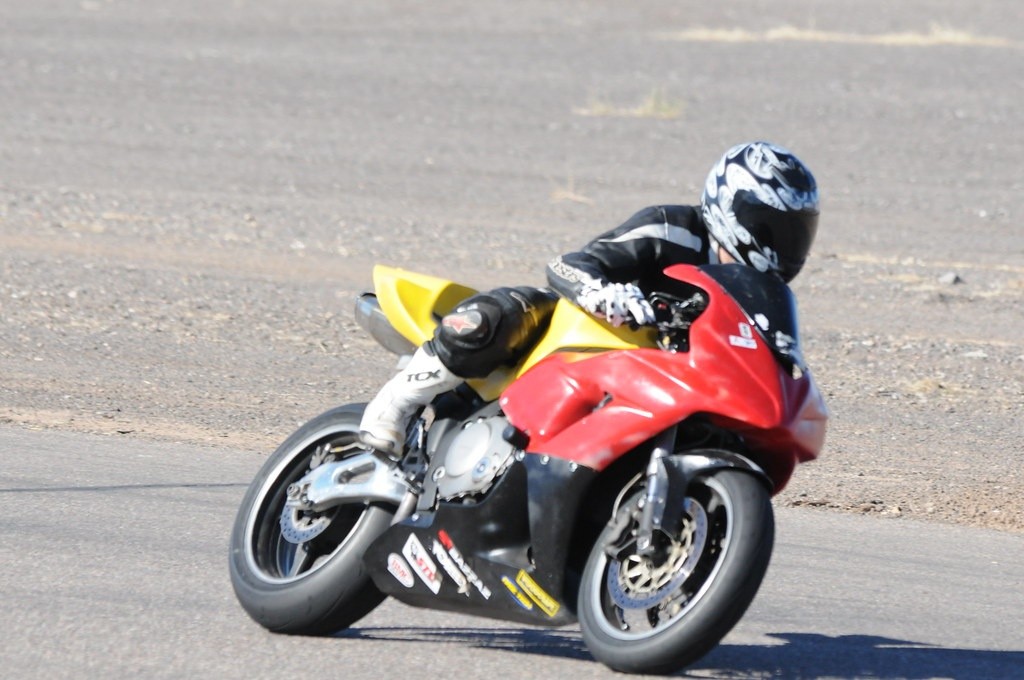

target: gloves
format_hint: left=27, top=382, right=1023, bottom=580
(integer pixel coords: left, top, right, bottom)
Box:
left=584, top=282, right=654, bottom=327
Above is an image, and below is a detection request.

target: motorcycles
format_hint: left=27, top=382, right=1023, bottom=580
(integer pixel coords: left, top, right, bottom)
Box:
left=224, top=256, right=829, bottom=677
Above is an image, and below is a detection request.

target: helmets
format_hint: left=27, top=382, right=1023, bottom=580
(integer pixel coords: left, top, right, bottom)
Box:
left=700, top=142, right=819, bottom=284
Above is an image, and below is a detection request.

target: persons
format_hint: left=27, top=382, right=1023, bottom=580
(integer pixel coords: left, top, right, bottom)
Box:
left=357, top=139, right=823, bottom=462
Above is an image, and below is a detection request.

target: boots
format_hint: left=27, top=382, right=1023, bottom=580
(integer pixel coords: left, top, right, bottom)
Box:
left=359, top=341, right=466, bottom=452
